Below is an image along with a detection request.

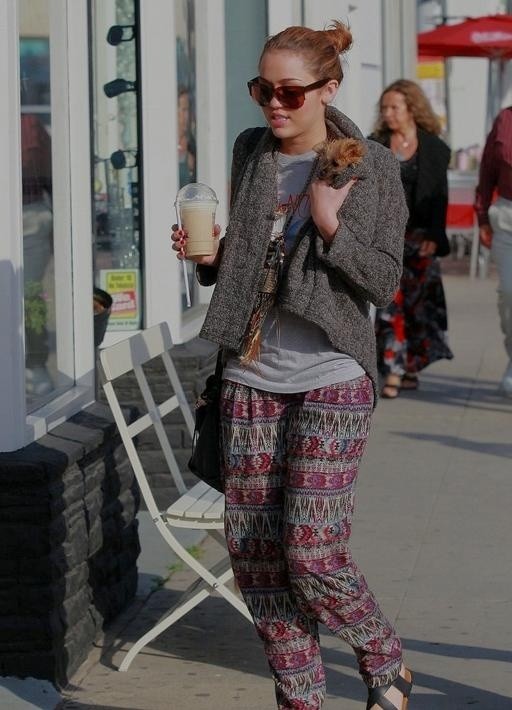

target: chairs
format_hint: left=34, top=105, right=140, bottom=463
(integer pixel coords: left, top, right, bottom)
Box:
left=443, top=171, right=488, bottom=278
left=88, top=323, right=257, bottom=669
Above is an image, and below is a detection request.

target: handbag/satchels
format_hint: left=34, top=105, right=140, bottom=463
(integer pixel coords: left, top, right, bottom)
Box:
left=187, top=375, right=224, bottom=493
left=259, top=240, right=281, bottom=294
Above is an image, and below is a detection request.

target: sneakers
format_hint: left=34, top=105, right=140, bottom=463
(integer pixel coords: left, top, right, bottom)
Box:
left=500, top=368, right=512, bottom=392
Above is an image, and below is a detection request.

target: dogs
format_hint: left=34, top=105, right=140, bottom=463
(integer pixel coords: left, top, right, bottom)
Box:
left=312, top=136, right=363, bottom=181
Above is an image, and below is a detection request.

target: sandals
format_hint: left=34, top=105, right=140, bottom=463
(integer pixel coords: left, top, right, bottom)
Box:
left=366, top=668, right=412, bottom=710
left=381, top=373, right=417, bottom=398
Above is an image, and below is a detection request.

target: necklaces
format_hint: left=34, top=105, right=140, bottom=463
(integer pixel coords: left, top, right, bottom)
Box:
left=236, top=150, right=321, bottom=370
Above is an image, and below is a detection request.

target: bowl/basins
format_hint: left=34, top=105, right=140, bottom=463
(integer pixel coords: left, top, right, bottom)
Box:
left=93, top=287, right=112, bottom=347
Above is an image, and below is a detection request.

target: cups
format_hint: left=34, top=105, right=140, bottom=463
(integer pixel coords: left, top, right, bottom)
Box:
left=175, top=181, right=220, bottom=256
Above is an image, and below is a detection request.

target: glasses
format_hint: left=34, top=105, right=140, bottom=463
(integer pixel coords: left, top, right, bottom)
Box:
left=247, top=77, right=332, bottom=109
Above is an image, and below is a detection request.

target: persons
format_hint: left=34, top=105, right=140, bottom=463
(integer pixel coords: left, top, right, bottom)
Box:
left=365, top=79, right=453, bottom=398
left=471, top=105, right=512, bottom=400
left=173, top=16, right=414, bottom=709
left=177, top=85, right=195, bottom=189
